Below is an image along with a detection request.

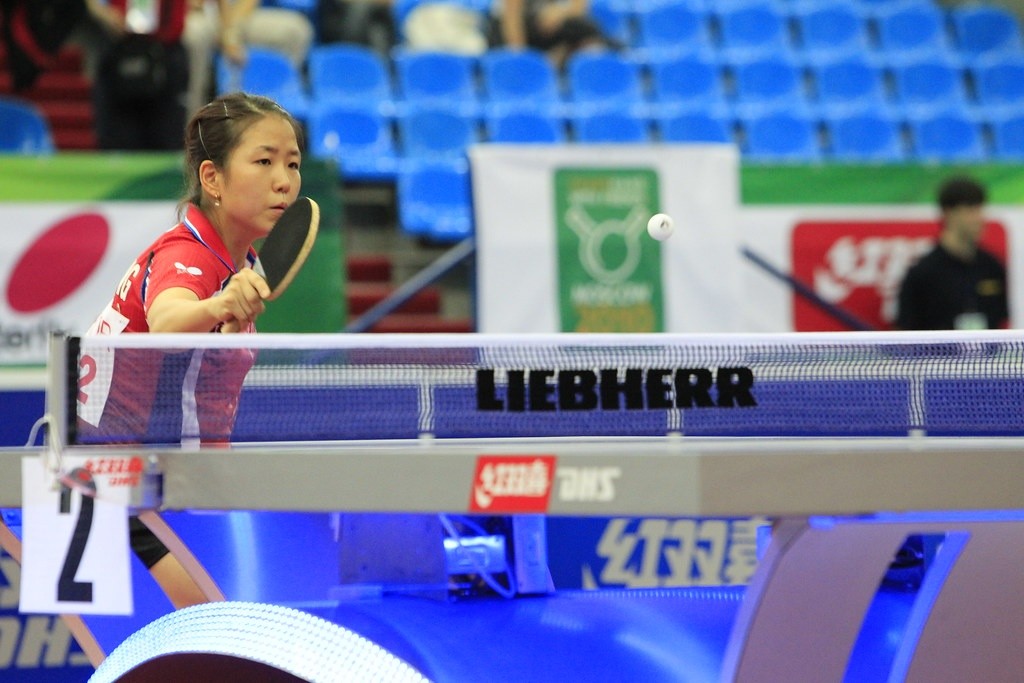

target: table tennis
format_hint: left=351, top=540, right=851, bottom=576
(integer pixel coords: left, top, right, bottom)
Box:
left=647, top=213, right=674, bottom=241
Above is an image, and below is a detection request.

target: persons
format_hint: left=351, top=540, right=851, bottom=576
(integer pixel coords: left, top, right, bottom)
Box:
left=76, top=93, right=303, bottom=612
left=8, top=0, right=606, bottom=91
left=897, top=174, right=1010, bottom=357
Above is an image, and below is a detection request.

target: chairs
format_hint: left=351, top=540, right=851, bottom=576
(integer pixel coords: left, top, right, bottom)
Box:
left=0, top=98, right=54, bottom=156
left=213, top=0, right=1024, bottom=242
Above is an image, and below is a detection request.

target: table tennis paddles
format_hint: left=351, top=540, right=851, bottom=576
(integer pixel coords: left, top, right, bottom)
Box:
left=218, top=196, right=323, bottom=334
